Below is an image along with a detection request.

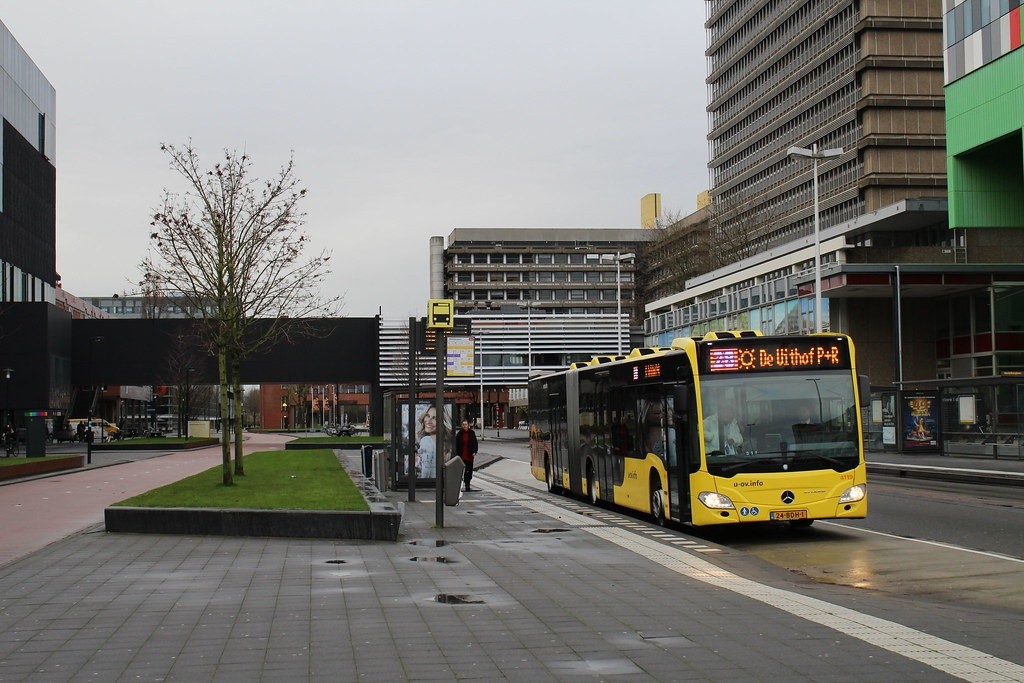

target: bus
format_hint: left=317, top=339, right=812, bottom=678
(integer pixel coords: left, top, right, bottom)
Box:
left=527, top=329, right=873, bottom=533
left=46, top=417, right=119, bottom=440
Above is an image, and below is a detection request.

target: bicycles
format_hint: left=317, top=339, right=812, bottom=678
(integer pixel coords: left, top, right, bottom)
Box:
left=0, top=436, right=19, bottom=458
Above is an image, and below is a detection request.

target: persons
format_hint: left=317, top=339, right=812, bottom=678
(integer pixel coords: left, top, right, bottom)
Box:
left=620, top=416, right=629, bottom=450
left=797, top=406, right=825, bottom=431
left=417, top=403, right=452, bottom=477
left=456, top=419, right=478, bottom=490
left=703, top=404, right=743, bottom=454
left=77, top=421, right=86, bottom=433
left=913, top=417, right=928, bottom=437
left=66, top=421, right=71, bottom=430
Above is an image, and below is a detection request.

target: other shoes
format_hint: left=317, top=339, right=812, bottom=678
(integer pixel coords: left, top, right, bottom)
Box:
left=466, top=485, right=471, bottom=490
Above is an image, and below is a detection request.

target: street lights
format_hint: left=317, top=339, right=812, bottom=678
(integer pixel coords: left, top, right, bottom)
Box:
left=617, top=251, right=636, bottom=358
left=787, top=143, right=843, bottom=335
left=471, top=329, right=491, bottom=440
left=185, top=366, right=196, bottom=442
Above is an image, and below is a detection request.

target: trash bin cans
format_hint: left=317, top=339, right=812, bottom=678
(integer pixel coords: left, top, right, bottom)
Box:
left=361, top=445, right=373, bottom=477
left=443, top=455, right=466, bottom=506
